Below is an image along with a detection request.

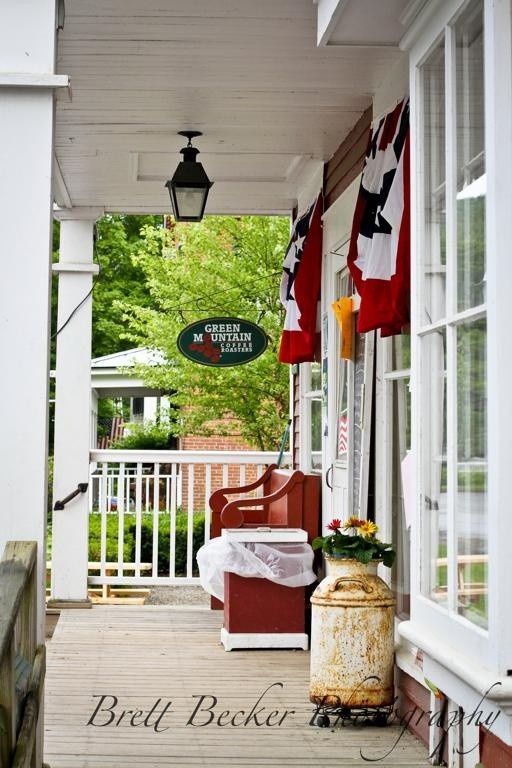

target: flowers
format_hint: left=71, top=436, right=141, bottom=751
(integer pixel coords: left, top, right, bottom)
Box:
left=311, top=514, right=398, bottom=571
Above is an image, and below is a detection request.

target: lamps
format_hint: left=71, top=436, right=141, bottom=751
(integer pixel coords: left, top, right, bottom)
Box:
left=164, top=129, right=214, bottom=222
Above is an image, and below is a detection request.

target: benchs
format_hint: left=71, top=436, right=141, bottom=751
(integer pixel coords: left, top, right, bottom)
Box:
left=204, top=464, right=316, bottom=654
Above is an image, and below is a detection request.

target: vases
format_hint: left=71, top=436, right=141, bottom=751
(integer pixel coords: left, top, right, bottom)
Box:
left=310, top=556, right=398, bottom=706
